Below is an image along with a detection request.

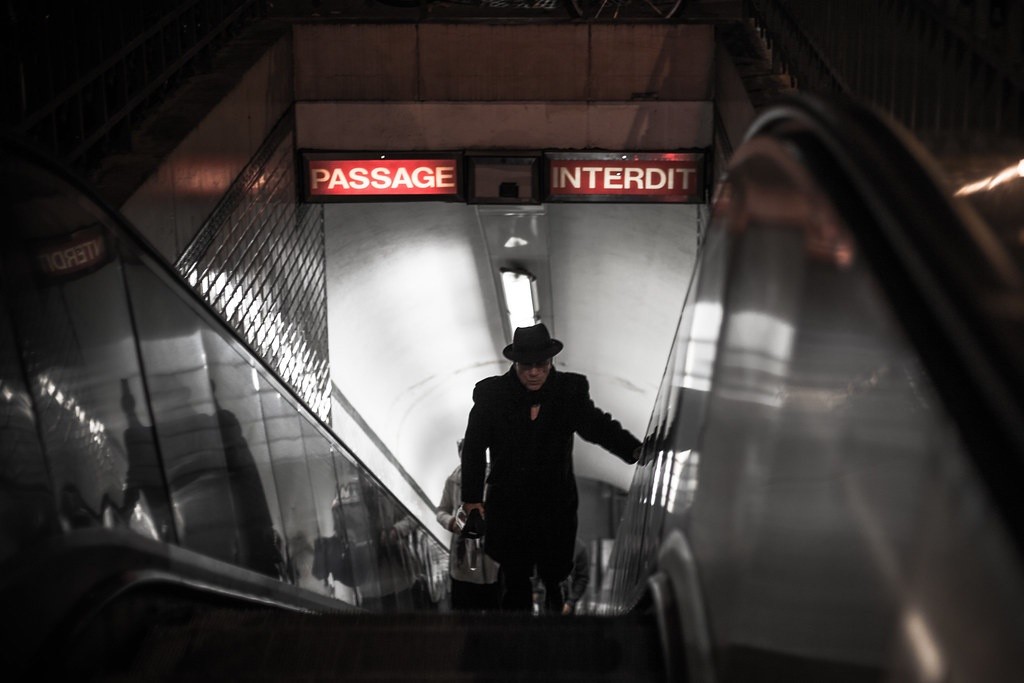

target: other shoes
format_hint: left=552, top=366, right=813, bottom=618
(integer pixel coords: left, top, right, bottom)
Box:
left=544, top=582, right=563, bottom=609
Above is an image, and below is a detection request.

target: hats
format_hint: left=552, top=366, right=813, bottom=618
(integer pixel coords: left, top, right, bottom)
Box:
left=502, top=323, right=564, bottom=364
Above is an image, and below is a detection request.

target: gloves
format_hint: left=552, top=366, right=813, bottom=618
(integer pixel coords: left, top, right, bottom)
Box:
left=460, top=501, right=485, bottom=538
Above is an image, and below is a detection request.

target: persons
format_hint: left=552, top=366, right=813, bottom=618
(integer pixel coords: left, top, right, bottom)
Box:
left=437, top=438, right=498, bottom=612
left=533, top=538, right=590, bottom=613
left=311, top=467, right=430, bottom=614
left=116, top=391, right=292, bottom=584
left=460, top=323, right=644, bottom=613
